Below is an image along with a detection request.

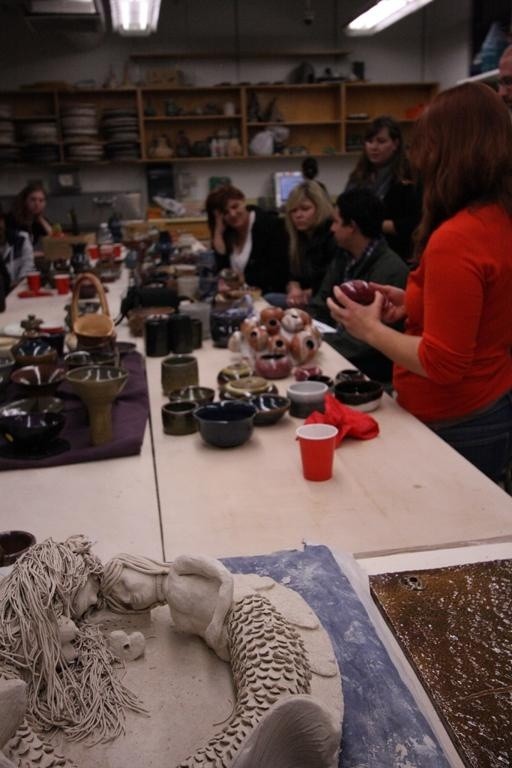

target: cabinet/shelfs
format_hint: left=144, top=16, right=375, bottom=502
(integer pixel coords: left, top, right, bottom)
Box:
left=0, top=80, right=443, bottom=168
left=115, top=212, right=212, bottom=247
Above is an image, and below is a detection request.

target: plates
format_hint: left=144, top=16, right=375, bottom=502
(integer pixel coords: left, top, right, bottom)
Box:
left=218, top=80, right=286, bottom=86
left=345, top=114, right=369, bottom=120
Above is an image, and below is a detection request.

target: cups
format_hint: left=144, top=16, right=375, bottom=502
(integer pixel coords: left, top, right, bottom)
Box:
left=134, top=268, right=247, bottom=357
left=25, top=271, right=43, bottom=295
left=53, top=272, right=72, bottom=295
left=223, top=102, right=236, bottom=116
left=89, top=244, right=124, bottom=259
left=295, top=423, right=339, bottom=484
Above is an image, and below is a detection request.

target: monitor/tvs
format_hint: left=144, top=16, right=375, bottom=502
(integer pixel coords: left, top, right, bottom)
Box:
left=271, top=170, right=303, bottom=209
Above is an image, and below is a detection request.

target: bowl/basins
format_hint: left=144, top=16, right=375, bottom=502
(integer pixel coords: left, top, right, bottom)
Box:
left=36, top=80, right=71, bottom=98
left=2, top=529, right=36, bottom=566
left=0, top=105, right=142, bottom=162
left=156, top=351, right=386, bottom=449
left=33, top=254, right=91, bottom=273
left=3, top=332, right=136, bottom=463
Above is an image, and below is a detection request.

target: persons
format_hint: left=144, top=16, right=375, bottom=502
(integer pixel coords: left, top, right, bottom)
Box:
left=496, top=44, right=512, bottom=105
left=304, top=189, right=411, bottom=393
left=0, top=200, right=38, bottom=280
left=327, top=82, right=512, bottom=484
left=338, top=116, right=419, bottom=260
left=6, top=185, right=54, bottom=245
left=263, top=179, right=338, bottom=306
left=205, top=185, right=288, bottom=295
left=302, top=155, right=327, bottom=193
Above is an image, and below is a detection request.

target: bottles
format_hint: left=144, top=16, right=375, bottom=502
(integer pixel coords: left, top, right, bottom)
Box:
left=100, top=222, right=115, bottom=272
left=107, top=61, right=131, bottom=86
left=208, top=128, right=244, bottom=158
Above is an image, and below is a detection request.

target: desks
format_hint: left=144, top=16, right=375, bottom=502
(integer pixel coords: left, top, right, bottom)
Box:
left=2, top=262, right=510, bottom=766
